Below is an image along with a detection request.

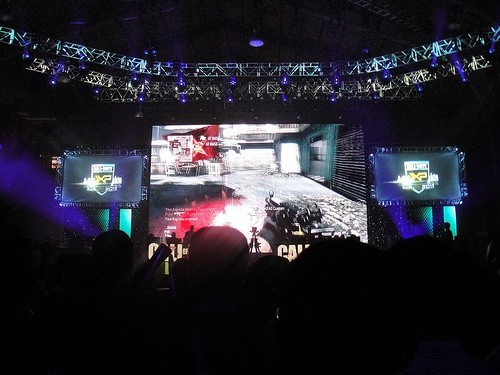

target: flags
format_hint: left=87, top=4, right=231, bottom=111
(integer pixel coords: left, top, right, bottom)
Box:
left=162, top=125, right=219, bottom=163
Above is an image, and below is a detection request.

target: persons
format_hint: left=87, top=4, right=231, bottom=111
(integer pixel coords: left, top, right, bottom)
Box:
left=0, top=189, right=500, bottom=375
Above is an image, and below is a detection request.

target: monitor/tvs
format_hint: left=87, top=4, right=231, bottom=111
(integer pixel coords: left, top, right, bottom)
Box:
left=366, top=146, right=469, bottom=207
left=56, top=147, right=151, bottom=209
